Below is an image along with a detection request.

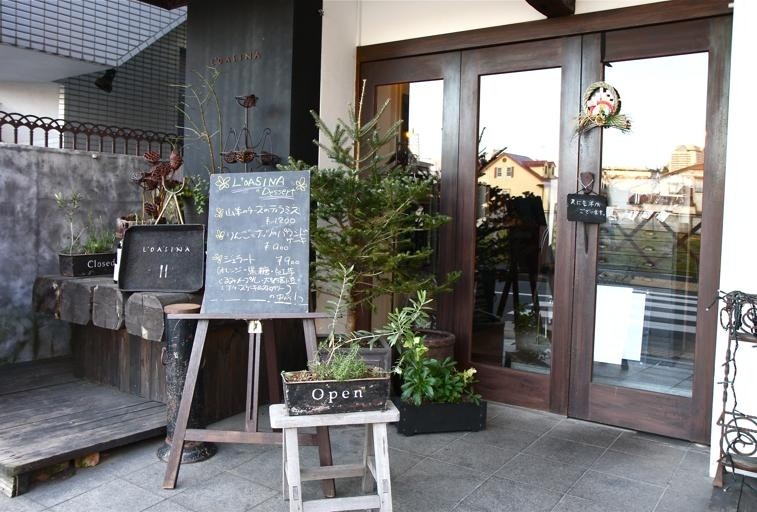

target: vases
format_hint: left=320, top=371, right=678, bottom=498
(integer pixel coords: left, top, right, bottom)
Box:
left=471, top=308, right=505, bottom=367
left=117, top=213, right=167, bottom=234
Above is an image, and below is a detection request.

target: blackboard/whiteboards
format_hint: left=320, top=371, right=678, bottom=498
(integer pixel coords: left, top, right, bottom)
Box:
left=203, top=170, right=311, bottom=313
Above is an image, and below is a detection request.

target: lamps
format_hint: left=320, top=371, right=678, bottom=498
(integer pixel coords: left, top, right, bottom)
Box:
left=95, top=69, right=117, bottom=93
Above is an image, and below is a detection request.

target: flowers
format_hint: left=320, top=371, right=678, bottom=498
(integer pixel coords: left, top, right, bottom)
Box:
left=595, top=114, right=605, bottom=127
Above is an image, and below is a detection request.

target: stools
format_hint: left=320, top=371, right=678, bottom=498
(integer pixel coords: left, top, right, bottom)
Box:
left=269, top=400, right=400, bottom=512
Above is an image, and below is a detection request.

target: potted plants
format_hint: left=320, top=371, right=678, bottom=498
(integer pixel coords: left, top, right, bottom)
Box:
left=54, top=191, right=117, bottom=277
left=512, top=290, right=541, bottom=362
left=276, top=80, right=487, bottom=436
